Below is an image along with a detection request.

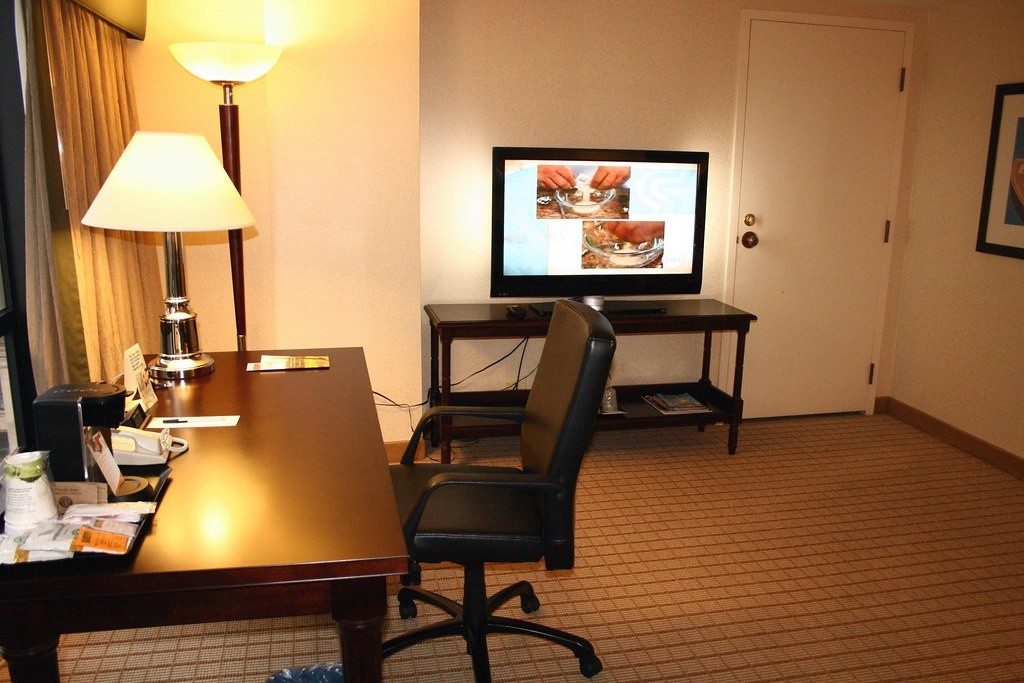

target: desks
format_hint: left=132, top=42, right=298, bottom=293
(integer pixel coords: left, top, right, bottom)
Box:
left=1, top=344, right=409, bottom=683
left=421, top=297, right=756, bottom=464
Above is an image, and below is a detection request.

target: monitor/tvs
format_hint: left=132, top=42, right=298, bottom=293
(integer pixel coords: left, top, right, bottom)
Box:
left=489, top=146, right=710, bottom=313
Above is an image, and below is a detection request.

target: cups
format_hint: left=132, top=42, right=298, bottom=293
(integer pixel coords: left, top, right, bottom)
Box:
left=602, top=388, right=617, bottom=413
left=4, top=451, right=59, bottom=536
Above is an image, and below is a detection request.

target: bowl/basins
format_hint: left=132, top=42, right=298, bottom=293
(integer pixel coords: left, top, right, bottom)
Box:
left=582, top=221, right=664, bottom=267
left=554, top=184, right=617, bottom=216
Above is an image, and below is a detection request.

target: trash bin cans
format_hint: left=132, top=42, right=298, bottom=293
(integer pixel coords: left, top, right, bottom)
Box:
left=265, top=664, right=344, bottom=683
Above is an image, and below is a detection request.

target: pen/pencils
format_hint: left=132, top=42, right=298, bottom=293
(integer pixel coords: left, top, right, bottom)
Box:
left=162, top=419, right=223, bottom=423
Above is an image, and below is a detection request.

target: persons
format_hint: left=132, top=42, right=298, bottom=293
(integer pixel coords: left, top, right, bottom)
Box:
left=607, top=222, right=664, bottom=244
left=538, top=165, right=631, bottom=191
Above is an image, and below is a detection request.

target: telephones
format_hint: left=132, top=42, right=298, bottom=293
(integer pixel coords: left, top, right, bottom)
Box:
left=111, top=425, right=173, bottom=465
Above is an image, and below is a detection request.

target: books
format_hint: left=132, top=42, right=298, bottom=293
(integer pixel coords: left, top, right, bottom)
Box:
left=641, top=390, right=712, bottom=414
left=246, top=354, right=330, bottom=372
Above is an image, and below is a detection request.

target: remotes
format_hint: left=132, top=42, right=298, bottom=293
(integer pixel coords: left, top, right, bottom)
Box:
left=505, top=304, right=527, bottom=319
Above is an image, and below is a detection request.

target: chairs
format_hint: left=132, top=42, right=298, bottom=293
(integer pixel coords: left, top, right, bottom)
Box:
left=379, top=299, right=617, bottom=683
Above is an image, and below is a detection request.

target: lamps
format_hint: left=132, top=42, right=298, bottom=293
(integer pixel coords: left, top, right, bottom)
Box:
left=167, top=40, right=286, bottom=353
left=79, top=127, right=256, bottom=382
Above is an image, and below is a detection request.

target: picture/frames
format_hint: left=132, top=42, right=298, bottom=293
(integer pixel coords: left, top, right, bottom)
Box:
left=975, top=83, right=1024, bottom=261
